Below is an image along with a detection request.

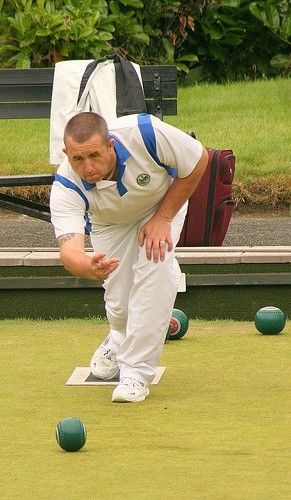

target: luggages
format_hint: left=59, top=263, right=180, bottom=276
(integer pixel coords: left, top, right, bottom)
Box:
left=177, top=129, right=236, bottom=247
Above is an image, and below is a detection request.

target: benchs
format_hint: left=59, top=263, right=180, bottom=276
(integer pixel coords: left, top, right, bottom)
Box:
left=0, top=58, right=177, bottom=236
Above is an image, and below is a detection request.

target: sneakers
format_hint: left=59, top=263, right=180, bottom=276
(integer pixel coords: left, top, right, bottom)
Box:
left=91, top=333, right=120, bottom=380
left=111, top=377, right=149, bottom=402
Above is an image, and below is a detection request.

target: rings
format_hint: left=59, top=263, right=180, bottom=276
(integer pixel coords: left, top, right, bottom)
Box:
left=159, top=240, right=165, bottom=243
left=98, top=263, right=103, bottom=269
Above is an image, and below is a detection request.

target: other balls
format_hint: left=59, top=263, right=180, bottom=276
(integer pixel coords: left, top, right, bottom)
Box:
left=166, top=308, right=189, bottom=340
left=54, top=416, right=88, bottom=452
left=253, top=305, right=287, bottom=336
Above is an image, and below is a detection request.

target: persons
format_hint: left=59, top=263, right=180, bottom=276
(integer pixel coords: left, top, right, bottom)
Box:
left=50, top=112, right=209, bottom=402
